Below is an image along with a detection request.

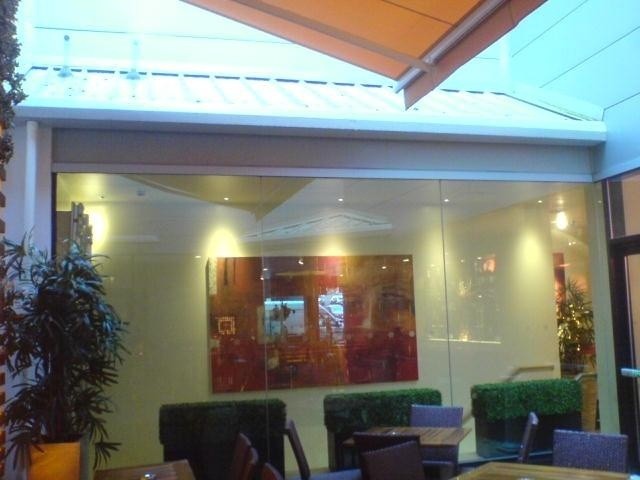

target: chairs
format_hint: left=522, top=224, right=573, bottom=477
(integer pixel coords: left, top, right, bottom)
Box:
left=259, top=462, right=281, bottom=479
left=227, top=432, right=259, bottom=479
left=358, top=440, right=425, bottom=479
left=518, top=412, right=540, bottom=464
left=285, top=419, right=311, bottom=480
left=551, top=429, right=629, bottom=474
left=352, top=430, right=420, bottom=452
left=410, top=404, right=463, bottom=430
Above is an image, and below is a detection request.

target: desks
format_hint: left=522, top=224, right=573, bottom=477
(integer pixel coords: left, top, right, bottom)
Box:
left=451, top=461, right=640, bottom=480
left=94, top=459, right=195, bottom=480
left=342, top=426, right=472, bottom=476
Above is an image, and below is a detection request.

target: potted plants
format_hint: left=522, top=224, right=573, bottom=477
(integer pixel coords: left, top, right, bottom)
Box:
left=0, top=223, right=130, bottom=479
